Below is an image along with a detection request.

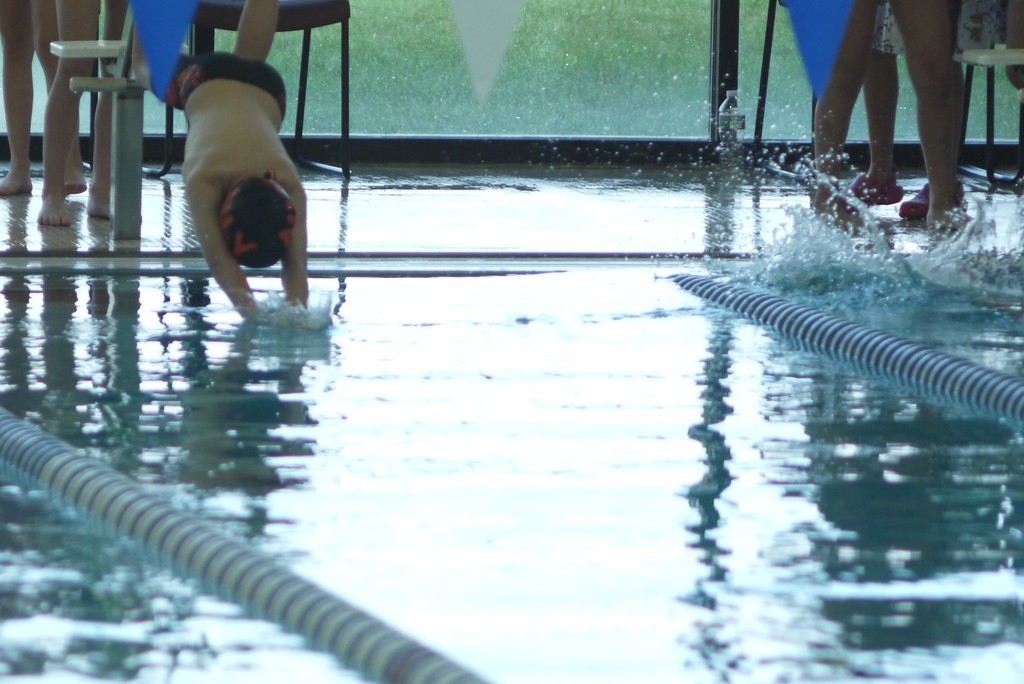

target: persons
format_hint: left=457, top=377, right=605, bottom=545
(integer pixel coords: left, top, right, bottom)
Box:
left=0, top=0, right=308, bottom=318
left=812, top=0, right=1024, bottom=237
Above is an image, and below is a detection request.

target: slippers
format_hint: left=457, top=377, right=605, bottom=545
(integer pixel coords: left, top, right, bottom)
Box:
left=843, top=172, right=904, bottom=211
left=900, top=179, right=968, bottom=218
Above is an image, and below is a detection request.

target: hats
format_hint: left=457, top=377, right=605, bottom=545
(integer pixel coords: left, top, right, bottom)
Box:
left=221, top=177, right=296, bottom=269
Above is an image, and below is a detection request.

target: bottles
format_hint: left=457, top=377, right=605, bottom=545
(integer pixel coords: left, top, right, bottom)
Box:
left=717, top=90, right=746, bottom=170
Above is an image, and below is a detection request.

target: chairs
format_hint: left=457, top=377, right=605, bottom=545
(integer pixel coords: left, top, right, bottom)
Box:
left=92, top=1, right=351, bottom=179
